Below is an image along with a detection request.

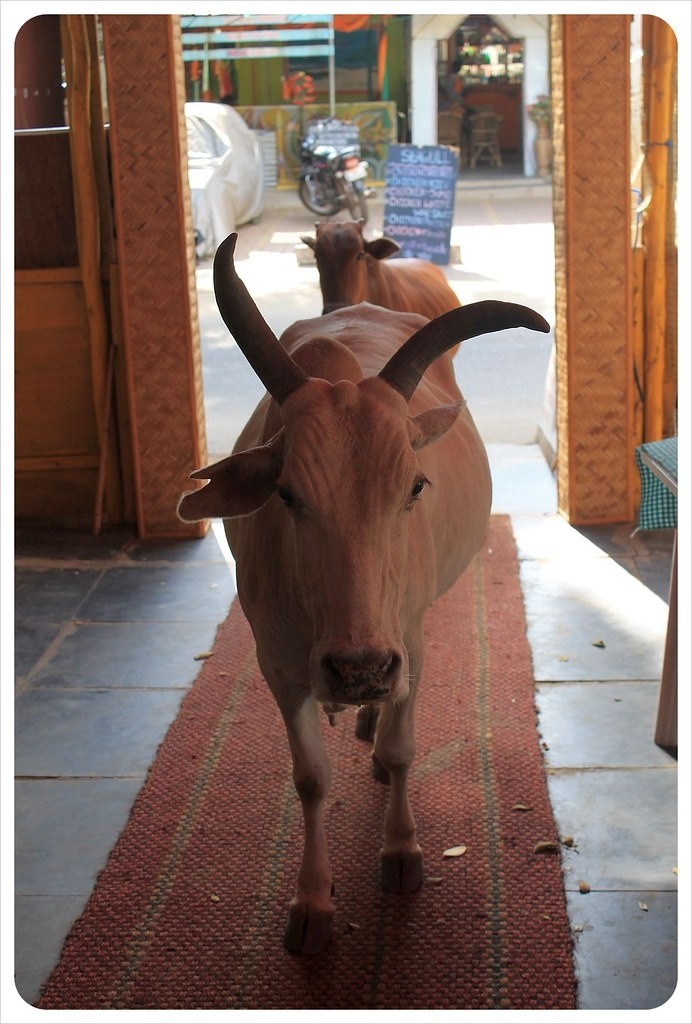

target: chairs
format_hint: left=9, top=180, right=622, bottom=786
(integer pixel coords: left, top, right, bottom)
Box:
left=468, top=112, right=504, bottom=170
left=437, top=111, right=466, bottom=172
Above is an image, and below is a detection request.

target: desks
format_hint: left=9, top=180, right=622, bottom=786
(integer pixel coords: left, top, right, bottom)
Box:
left=635, top=433, right=679, bottom=749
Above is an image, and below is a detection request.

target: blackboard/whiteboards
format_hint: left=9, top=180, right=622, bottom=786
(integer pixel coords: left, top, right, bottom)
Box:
left=384, top=143, right=460, bottom=264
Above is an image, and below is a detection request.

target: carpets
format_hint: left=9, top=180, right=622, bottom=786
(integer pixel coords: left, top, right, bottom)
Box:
left=30, top=514, right=578, bottom=1010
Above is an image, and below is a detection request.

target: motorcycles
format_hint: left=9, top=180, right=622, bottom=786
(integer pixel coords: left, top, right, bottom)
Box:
left=298, top=115, right=377, bottom=229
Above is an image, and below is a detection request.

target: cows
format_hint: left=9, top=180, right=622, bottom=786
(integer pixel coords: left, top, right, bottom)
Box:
left=299, top=218, right=464, bottom=362
left=177, top=231, right=551, bottom=962
